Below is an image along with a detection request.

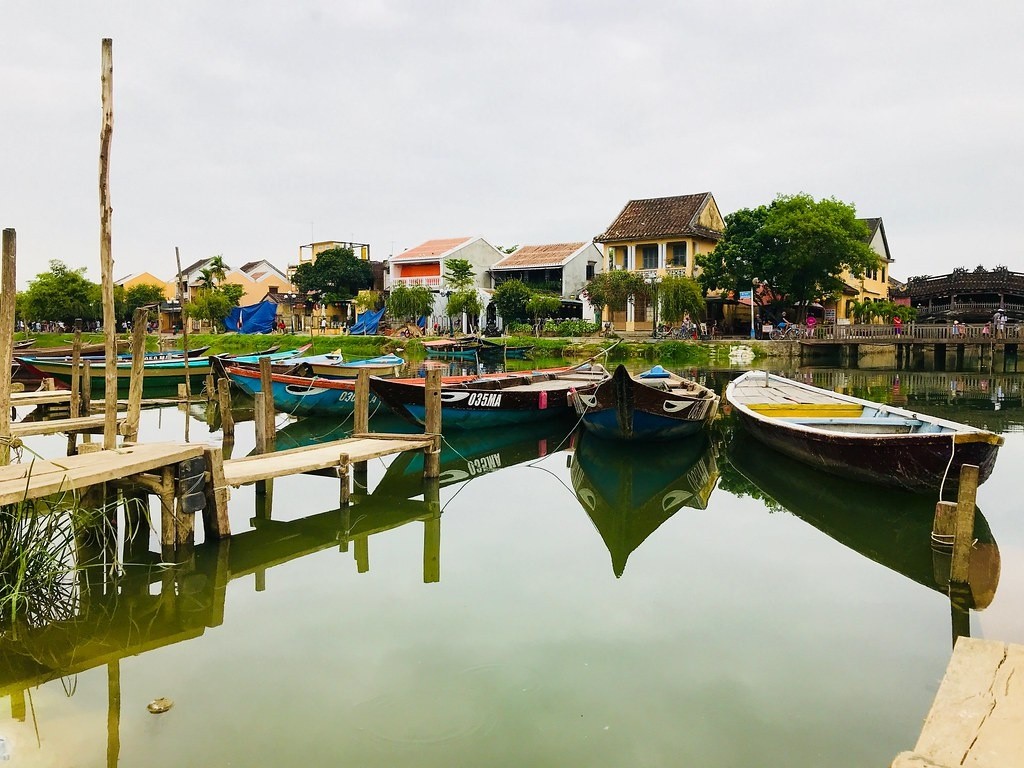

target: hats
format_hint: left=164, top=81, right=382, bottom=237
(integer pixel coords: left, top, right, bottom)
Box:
left=954, top=320, right=959, bottom=325
left=127, top=321, right=131, bottom=324
left=998, top=308, right=1004, bottom=312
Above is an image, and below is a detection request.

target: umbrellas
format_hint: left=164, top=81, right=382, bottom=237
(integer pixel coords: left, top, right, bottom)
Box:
left=794, top=300, right=825, bottom=318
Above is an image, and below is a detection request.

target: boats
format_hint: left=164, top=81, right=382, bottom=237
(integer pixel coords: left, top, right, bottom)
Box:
left=571, top=426, right=721, bottom=579
left=727, top=369, right=1005, bottom=496
left=721, top=413, right=1001, bottom=611
left=369, top=427, right=574, bottom=502
left=10, top=329, right=724, bottom=421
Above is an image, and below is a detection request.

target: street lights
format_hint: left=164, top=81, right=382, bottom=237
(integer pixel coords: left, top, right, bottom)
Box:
left=440, top=284, right=458, bottom=337
left=167, top=297, right=179, bottom=335
left=644, top=272, right=662, bottom=340
left=284, top=290, right=296, bottom=335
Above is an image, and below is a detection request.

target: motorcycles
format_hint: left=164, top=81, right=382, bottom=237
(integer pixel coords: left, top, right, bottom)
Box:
left=481, top=319, right=501, bottom=337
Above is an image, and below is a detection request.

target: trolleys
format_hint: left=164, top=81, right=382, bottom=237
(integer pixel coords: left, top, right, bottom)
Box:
left=404, top=320, right=423, bottom=338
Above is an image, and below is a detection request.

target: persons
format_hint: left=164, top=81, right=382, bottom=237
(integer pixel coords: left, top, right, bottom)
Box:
left=14, top=318, right=100, bottom=333
left=146, top=320, right=157, bottom=333
left=172, top=323, right=179, bottom=335
left=993, top=309, right=1007, bottom=339
left=806, top=313, right=816, bottom=337
left=982, top=323, right=989, bottom=337
left=321, top=318, right=326, bottom=335
left=952, top=320, right=966, bottom=339
left=893, top=315, right=901, bottom=339
left=279, top=320, right=286, bottom=333
left=777, top=312, right=789, bottom=340
left=271, top=319, right=277, bottom=331
left=121, top=320, right=133, bottom=333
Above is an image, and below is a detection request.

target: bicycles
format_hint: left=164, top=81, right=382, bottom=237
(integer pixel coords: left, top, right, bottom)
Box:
left=603, top=320, right=612, bottom=338
left=769, top=322, right=802, bottom=341
left=653, top=320, right=723, bottom=340
